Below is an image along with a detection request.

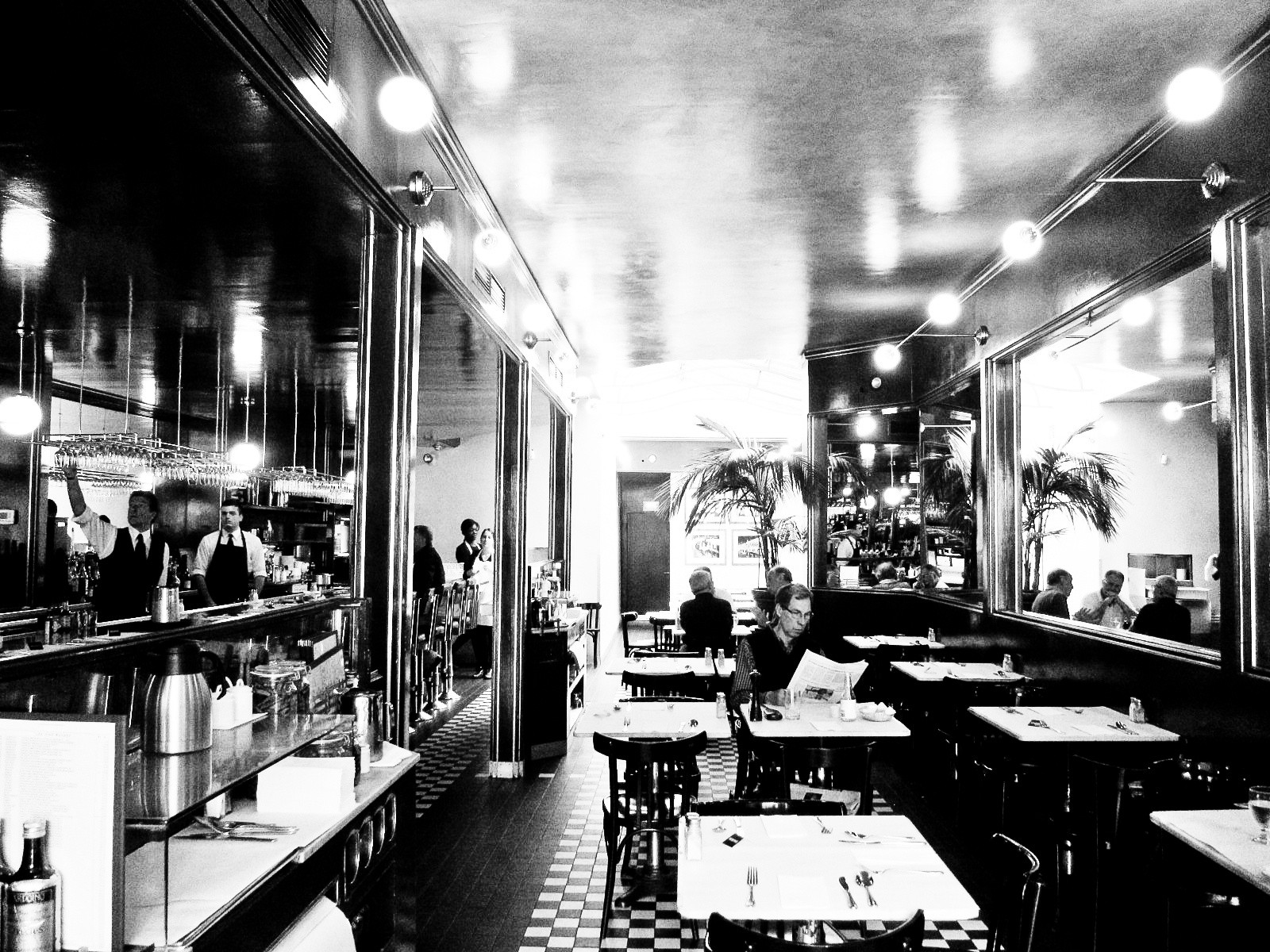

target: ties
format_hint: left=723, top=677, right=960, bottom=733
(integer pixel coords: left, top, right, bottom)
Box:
left=134, top=534, right=147, bottom=561
left=226, top=535, right=234, bottom=546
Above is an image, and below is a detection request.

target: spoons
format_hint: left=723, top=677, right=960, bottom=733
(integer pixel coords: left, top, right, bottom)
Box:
left=193, top=815, right=297, bottom=830
left=855, top=871, right=879, bottom=907
left=1063, top=707, right=1083, bottom=713
left=1116, top=721, right=1139, bottom=735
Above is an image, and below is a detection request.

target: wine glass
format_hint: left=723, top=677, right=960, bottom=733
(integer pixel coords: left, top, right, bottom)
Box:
left=1248, top=786, right=1270, bottom=844
left=54, top=435, right=354, bottom=498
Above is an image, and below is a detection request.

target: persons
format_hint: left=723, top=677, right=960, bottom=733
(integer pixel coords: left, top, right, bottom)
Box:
left=875, top=559, right=910, bottom=588
left=191, top=499, right=267, bottom=621
left=915, top=565, right=949, bottom=589
left=98, top=515, right=110, bottom=523
left=413, top=524, right=445, bottom=633
left=454, top=519, right=493, bottom=679
left=1031, top=568, right=1073, bottom=621
left=1076, top=570, right=1136, bottom=631
left=57, top=456, right=169, bottom=633
left=1129, top=574, right=1191, bottom=645
left=837, top=520, right=864, bottom=578
left=47, top=501, right=57, bottom=566
left=734, top=583, right=858, bottom=704
left=679, top=566, right=734, bottom=658
left=749, top=565, right=793, bottom=625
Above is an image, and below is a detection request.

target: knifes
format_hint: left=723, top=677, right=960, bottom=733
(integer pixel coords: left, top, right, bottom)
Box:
left=1107, top=725, right=1130, bottom=735
left=172, top=834, right=276, bottom=843
left=839, top=876, right=858, bottom=909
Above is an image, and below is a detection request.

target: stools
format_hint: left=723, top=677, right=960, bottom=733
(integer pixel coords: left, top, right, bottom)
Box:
left=409, top=579, right=480, bottom=734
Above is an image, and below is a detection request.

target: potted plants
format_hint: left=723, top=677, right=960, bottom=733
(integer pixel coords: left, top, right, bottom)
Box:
left=1021, top=421, right=1136, bottom=612
left=652, top=413, right=847, bottom=613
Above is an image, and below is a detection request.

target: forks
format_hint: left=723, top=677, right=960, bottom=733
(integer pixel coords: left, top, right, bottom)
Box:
left=204, top=818, right=298, bottom=834
left=746, top=816, right=832, bottom=907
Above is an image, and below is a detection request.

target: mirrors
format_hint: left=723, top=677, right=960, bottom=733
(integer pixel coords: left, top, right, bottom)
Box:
left=825, top=369, right=988, bottom=611
left=989, top=230, right=1225, bottom=670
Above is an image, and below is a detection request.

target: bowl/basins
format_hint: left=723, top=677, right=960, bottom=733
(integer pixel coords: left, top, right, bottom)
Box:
left=859, top=706, right=897, bottom=722
left=314, top=575, right=331, bottom=585
left=294, top=544, right=311, bottom=558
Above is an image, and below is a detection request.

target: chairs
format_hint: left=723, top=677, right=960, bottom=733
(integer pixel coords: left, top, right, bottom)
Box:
left=578, top=603, right=1270, bottom=952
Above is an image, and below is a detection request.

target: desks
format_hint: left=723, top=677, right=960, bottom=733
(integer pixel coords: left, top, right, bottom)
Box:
left=1148, top=810, right=1270, bottom=896
left=715, top=658, right=738, bottom=677
left=731, top=611, right=757, bottom=620
left=645, top=611, right=680, bottom=619
left=677, top=816, right=982, bottom=922
left=605, top=658, right=716, bottom=676
left=842, top=635, right=945, bottom=649
left=574, top=702, right=732, bottom=907
left=663, top=624, right=752, bottom=636
left=890, top=661, right=1025, bottom=682
left=966, top=706, right=1180, bottom=874
left=739, top=702, right=911, bottom=737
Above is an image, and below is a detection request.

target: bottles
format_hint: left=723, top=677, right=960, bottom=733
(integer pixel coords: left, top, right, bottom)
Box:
left=928, top=628, right=935, bottom=641
left=61, top=601, right=70, bottom=631
left=840, top=671, right=857, bottom=721
left=184, top=568, right=191, bottom=589
left=1128, top=698, right=1146, bottom=723
left=716, top=693, right=726, bottom=718
left=705, top=647, right=724, bottom=667
left=250, top=589, right=259, bottom=603
left=275, top=567, right=287, bottom=582
left=1002, top=654, right=1013, bottom=672
left=684, top=812, right=701, bottom=862
left=0, top=817, right=63, bottom=952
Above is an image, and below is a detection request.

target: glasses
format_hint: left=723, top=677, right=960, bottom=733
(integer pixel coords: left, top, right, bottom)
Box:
left=786, top=607, right=814, bottom=620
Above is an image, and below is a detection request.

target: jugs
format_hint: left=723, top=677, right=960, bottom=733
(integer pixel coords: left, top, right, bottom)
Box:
left=333, top=687, right=396, bottom=762
left=135, top=638, right=226, bottom=754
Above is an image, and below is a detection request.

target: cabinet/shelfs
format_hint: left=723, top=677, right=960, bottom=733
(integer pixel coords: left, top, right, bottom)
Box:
left=523, top=606, right=588, bottom=759
left=242, top=506, right=328, bottom=544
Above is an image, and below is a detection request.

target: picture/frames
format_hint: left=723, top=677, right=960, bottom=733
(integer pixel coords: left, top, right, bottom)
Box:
left=684, top=487, right=765, bottom=565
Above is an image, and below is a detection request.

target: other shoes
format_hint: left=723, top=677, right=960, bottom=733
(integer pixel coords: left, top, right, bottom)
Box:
left=483, top=668, right=492, bottom=679
left=472, top=666, right=484, bottom=679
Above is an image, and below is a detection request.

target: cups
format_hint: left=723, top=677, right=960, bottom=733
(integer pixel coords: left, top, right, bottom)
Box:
left=785, top=688, right=800, bottom=720
left=831, top=704, right=840, bottom=718
